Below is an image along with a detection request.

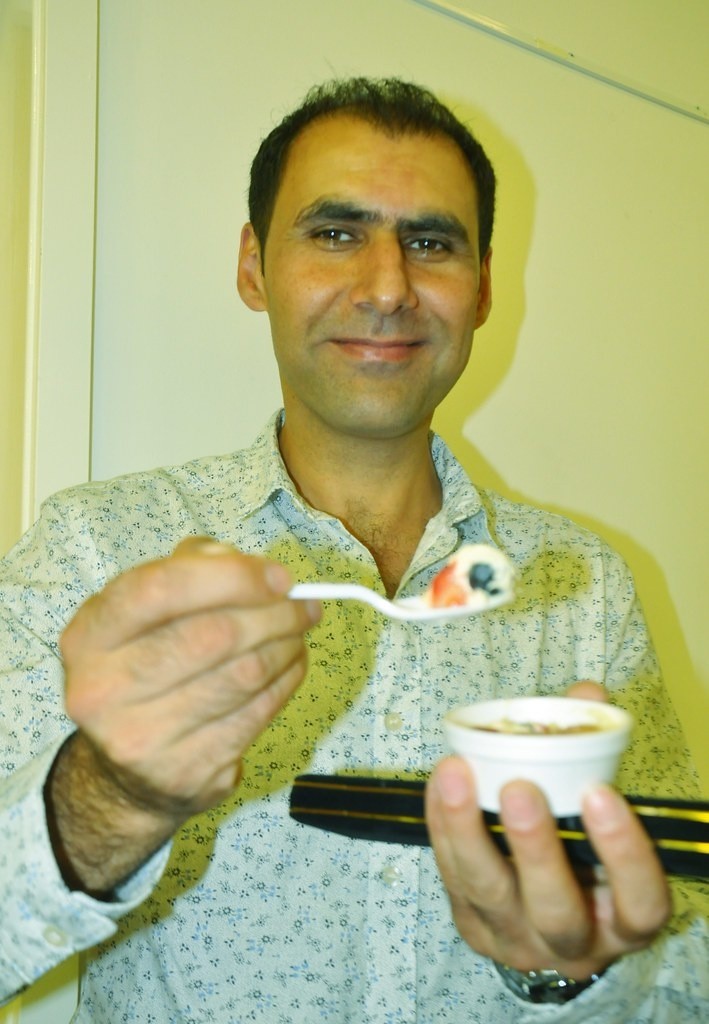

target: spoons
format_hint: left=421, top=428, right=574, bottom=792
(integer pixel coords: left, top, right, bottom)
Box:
left=291, top=576, right=513, bottom=625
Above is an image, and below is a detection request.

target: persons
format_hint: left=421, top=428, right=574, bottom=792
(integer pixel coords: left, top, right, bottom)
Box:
left=0, top=82, right=709, bottom=1024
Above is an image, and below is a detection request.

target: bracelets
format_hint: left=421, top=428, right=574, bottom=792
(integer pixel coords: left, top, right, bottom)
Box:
left=497, top=963, right=604, bottom=1004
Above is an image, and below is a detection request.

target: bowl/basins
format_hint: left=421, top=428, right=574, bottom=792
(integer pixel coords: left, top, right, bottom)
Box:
left=444, top=696, right=632, bottom=816
